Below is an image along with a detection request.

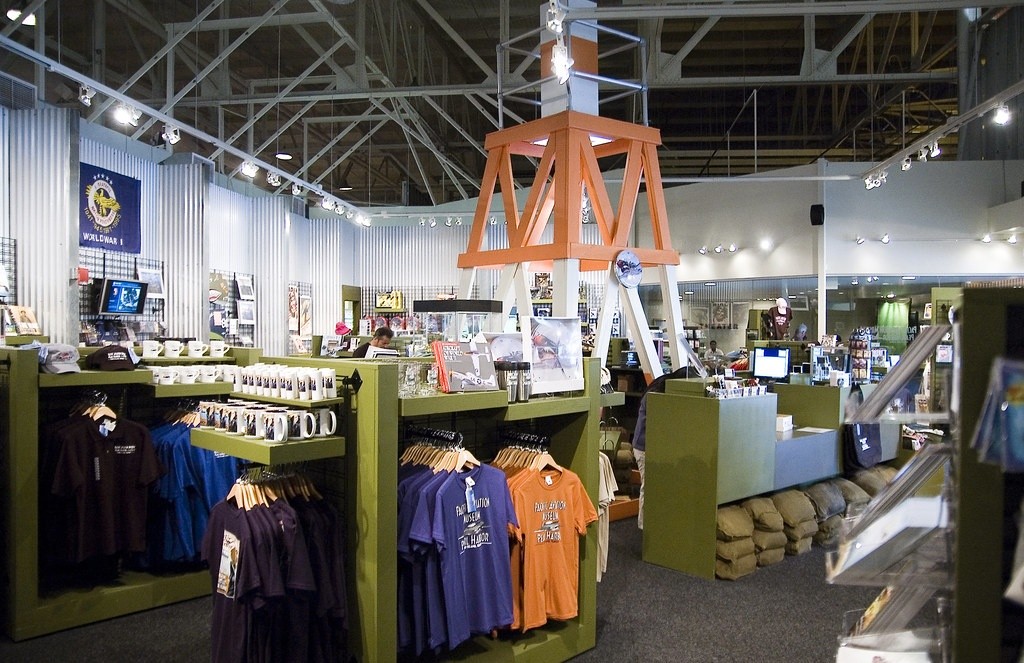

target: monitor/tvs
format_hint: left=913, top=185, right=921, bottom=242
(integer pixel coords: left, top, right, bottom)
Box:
left=752, top=346, right=791, bottom=383
left=621, top=351, right=641, bottom=369
left=98, top=278, right=149, bottom=316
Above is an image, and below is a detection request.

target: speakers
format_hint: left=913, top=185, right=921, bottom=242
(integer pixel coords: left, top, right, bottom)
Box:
left=810, top=204, right=824, bottom=225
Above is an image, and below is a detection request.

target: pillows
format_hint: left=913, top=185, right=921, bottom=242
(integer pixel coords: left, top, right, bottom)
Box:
left=784, top=536, right=812, bottom=555
left=716, top=504, right=755, bottom=542
left=818, top=512, right=844, bottom=532
left=831, top=476, right=873, bottom=504
left=846, top=465, right=888, bottom=498
left=812, top=526, right=844, bottom=548
left=801, top=480, right=846, bottom=522
left=782, top=518, right=819, bottom=541
left=874, top=464, right=899, bottom=484
left=754, top=547, right=785, bottom=565
left=767, top=487, right=817, bottom=527
left=751, top=528, right=787, bottom=551
left=716, top=536, right=755, bottom=563
left=714, top=553, right=756, bottom=581
left=740, top=496, right=785, bottom=533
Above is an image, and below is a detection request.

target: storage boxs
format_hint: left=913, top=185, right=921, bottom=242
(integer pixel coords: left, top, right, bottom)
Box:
left=776, top=413, right=792, bottom=431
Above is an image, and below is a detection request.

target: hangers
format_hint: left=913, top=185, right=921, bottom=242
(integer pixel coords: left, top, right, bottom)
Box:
left=397, top=423, right=481, bottom=474
left=66, top=386, right=117, bottom=421
left=225, top=460, right=323, bottom=511
left=161, top=396, right=225, bottom=428
left=492, top=429, right=563, bottom=473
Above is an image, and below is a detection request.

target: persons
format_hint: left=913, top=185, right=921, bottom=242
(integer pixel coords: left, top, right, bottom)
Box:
left=704, top=341, right=723, bottom=359
left=632, top=366, right=701, bottom=532
left=353, top=327, right=394, bottom=358
left=769, top=298, right=794, bottom=339
left=834, top=334, right=841, bottom=346
left=20, top=311, right=36, bottom=332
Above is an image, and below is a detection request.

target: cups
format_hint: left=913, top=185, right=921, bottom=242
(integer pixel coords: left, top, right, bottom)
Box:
left=139, top=340, right=238, bottom=386
left=196, top=398, right=337, bottom=445
left=493, top=361, right=531, bottom=404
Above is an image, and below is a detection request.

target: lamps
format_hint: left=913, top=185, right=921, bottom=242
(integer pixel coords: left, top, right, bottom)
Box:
left=928, top=140, right=941, bottom=158
left=714, top=244, right=724, bottom=254
left=698, top=244, right=709, bottom=256
left=418, top=216, right=426, bottom=226
left=444, top=216, right=452, bottom=228
left=992, top=102, right=1011, bottom=126
left=728, top=242, right=738, bottom=253
left=291, top=183, right=301, bottom=195
left=428, top=217, right=437, bottom=228
left=851, top=276, right=859, bottom=285
left=881, top=232, right=890, bottom=245
left=916, top=146, right=928, bottom=163
left=321, top=0, right=371, bottom=228
left=543, top=7, right=575, bottom=85
left=856, top=236, right=865, bottom=245
left=6, top=0, right=293, bottom=186
left=865, top=91, right=912, bottom=190
left=455, top=217, right=462, bottom=225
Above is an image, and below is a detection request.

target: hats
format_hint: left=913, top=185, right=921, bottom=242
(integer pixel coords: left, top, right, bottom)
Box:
left=86, top=344, right=141, bottom=373
left=39, top=343, right=82, bottom=373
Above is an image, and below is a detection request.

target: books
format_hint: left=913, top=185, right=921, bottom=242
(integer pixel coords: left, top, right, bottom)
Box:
left=9, top=306, right=42, bottom=336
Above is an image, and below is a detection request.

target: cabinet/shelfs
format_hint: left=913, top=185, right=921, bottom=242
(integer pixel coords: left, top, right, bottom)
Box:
left=188, top=354, right=625, bottom=663
left=640, top=274, right=1024, bottom=663
left=0, top=346, right=263, bottom=643
